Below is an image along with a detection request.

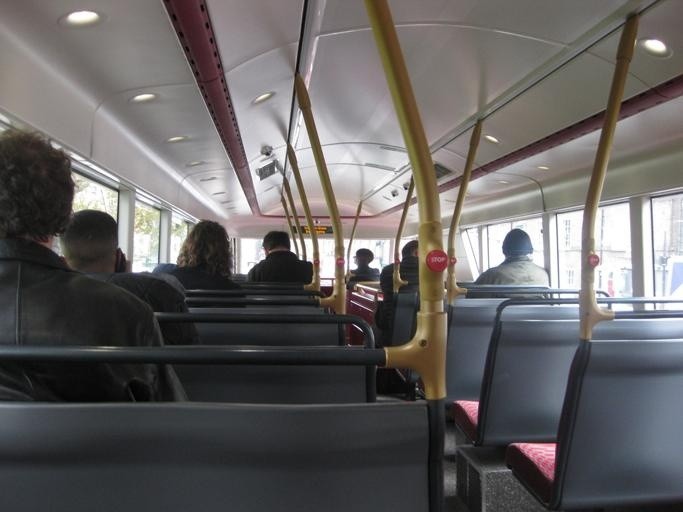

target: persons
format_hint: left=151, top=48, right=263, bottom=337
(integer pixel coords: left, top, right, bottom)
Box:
left=345, top=248, right=381, bottom=288
left=380, top=239, right=419, bottom=302
left=0, top=128, right=188, bottom=403
left=59, top=208, right=132, bottom=283
left=466, top=227, right=551, bottom=300
left=244, top=231, right=313, bottom=283
left=170, top=218, right=248, bottom=309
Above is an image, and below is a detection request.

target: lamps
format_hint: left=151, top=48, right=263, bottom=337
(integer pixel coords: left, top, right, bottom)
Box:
left=166, top=135, right=188, bottom=144
left=251, top=91, right=274, bottom=109
left=484, top=135, right=502, bottom=145
left=59, top=8, right=107, bottom=29
left=130, top=93, right=160, bottom=105
left=638, top=36, right=677, bottom=60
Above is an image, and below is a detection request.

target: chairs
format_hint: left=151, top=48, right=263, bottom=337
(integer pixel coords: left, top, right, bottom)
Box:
left=0, top=401, right=440, bottom=512
left=505, top=322, right=681, bottom=510
left=448, top=299, right=680, bottom=452
left=184, top=289, right=328, bottom=312
left=443, top=290, right=611, bottom=420
left=129, top=313, right=375, bottom=403
left=237, top=282, right=305, bottom=290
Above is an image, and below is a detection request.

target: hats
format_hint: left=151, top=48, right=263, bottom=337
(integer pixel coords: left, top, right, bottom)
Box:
left=502, top=229, right=533, bottom=254
